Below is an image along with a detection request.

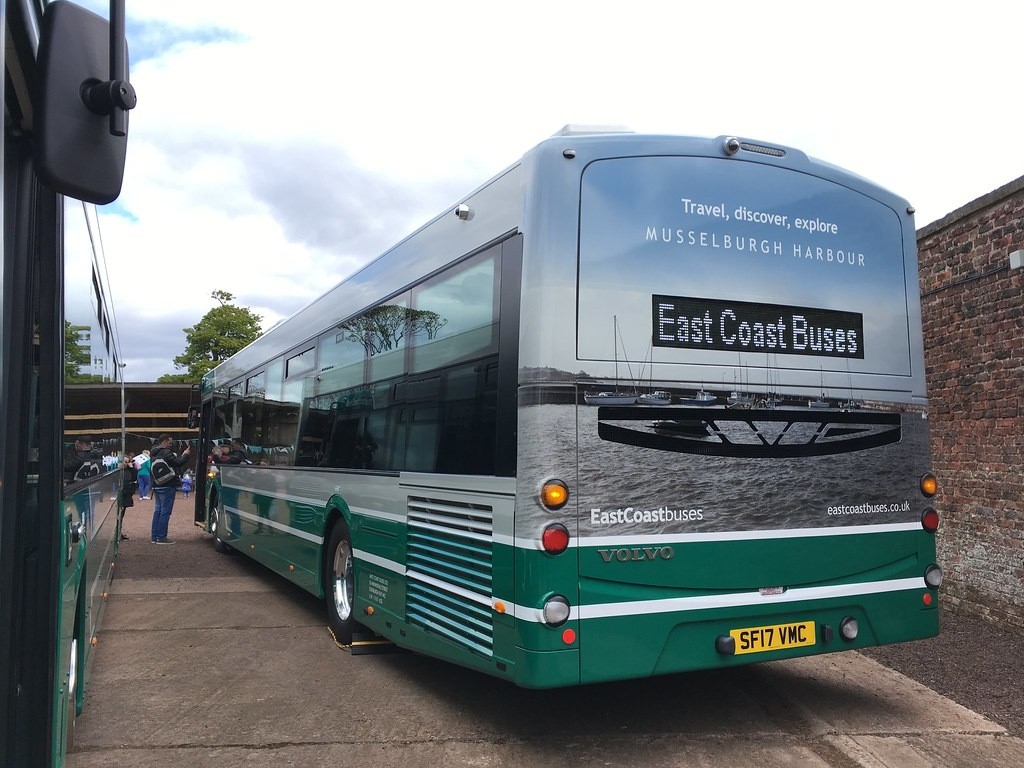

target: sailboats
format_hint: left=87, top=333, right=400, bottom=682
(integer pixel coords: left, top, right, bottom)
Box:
left=583, top=314, right=863, bottom=414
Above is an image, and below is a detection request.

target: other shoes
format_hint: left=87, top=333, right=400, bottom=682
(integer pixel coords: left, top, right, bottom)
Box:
left=140, top=495, right=151, bottom=500
left=222, top=531, right=241, bottom=542
left=121, top=534, right=130, bottom=541
left=267, top=529, right=275, bottom=536
left=150, top=538, right=176, bottom=544
left=252, top=528, right=264, bottom=534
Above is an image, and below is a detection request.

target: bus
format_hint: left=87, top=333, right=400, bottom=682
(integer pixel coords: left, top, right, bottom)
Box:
left=2, top=2, right=128, bottom=768
left=186, top=124, right=943, bottom=689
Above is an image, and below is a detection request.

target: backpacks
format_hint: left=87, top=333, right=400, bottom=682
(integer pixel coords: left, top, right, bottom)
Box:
left=150, top=448, right=175, bottom=485
left=226, top=450, right=252, bottom=483
left=73, top=455, right=102, bottom=483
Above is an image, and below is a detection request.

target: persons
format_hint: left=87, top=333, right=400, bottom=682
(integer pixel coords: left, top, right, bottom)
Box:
left=179, top=474, right=192, bottom=499
left=149, top=434, right=191, bottom=544
left=137, top=450, right=151, bottom=500
left=207, top=438, right=278, bottom=543
left=121, top=454, right=138, bottom=540
left=69, top=437, right=122, bottom=539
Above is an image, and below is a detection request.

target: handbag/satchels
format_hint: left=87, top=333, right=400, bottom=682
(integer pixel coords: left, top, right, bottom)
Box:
left=268, top=503, right=278, bottom=520
left=133, top=460, right=141, bottom=469
